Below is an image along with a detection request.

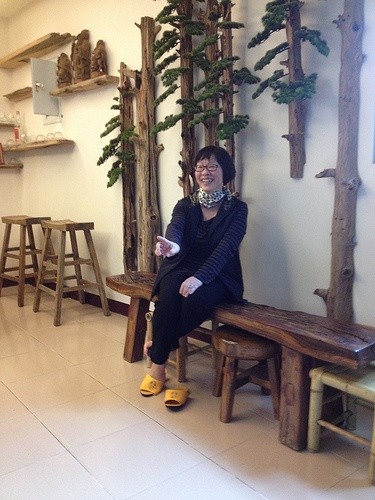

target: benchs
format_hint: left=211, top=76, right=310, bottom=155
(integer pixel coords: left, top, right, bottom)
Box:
left=105, top=271, right=375, bottom=452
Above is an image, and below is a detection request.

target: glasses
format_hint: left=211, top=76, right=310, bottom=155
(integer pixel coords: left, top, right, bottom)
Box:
left=193, top=164, right=220, bottom=171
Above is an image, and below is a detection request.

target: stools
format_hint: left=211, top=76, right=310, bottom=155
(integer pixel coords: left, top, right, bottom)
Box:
left=32, top=219, right=111, bottom=327
left=212, top=325, right=281, bottom=424
left=307, top=363, right=375, bottom=486
left=0, top=215, right=68, bottom=307
left=144, top=313, right=219, bottom=383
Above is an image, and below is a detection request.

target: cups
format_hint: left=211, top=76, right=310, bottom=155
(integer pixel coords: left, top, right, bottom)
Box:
left=1, top=130, right=68, bottom=147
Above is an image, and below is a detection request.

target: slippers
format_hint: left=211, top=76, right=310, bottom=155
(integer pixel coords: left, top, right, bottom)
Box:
left=139, top=368, right=167, bottom=397
left=165, top=386, right=189, bottom=407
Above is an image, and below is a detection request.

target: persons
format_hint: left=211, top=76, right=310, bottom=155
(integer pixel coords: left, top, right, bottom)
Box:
left=140, top=145, right=248, bottom=397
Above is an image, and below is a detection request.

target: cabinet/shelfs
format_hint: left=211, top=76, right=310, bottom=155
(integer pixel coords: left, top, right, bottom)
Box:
left=0, top=32, right=120, bottom=170
